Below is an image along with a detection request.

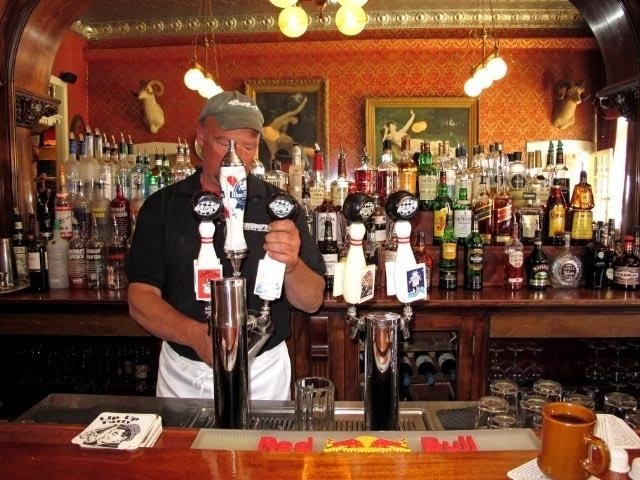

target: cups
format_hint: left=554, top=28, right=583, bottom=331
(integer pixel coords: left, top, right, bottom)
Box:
left=606, top=445, right=640, bottom=480
left=474, top=379, right=639, bottom=435
left=294, top=376, right=335, bottom=433
left=537, top=402, right=610, bottom=480
left=0, top=238, right=14, bottom=288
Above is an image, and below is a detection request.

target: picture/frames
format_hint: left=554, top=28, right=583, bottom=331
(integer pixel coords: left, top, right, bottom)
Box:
left=360, top=94, right=480, bottom=175
left=239, top=75, right=332, bottom=180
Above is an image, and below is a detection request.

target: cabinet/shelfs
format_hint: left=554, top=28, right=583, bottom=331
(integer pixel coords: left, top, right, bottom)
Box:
left=348, top=309, right=476, bottom=402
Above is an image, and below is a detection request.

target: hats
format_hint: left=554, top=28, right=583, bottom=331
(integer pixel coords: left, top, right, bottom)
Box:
left=197, top=89, right=268, bottom=137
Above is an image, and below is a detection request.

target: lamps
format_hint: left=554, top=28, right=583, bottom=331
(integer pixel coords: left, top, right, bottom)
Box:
left=181, top=0, right=228, bottom=100
left=272, top=0, right=374, bottom=44
left=462, top=0, right=507, bottom=102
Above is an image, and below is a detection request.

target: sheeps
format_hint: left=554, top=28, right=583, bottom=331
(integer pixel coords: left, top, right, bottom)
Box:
left=136, top=78, right=166, bottom=134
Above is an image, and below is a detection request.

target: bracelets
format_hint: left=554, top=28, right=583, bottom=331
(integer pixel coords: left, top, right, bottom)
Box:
left=284, top=259, right=300, bottom=274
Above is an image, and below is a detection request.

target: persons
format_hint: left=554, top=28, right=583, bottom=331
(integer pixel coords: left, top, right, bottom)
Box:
left=381, top=110, right=442, bottom=155
left=260, top=96, right=311, bottom=170
left=124, top=90, right=327, bottom=398
left=84, top=422, right=140, bottom=449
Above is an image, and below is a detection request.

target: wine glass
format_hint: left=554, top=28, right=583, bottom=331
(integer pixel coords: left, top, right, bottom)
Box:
left=487, top=341, right=546, bottom=383
left=586, top=339, right=639, bottom=391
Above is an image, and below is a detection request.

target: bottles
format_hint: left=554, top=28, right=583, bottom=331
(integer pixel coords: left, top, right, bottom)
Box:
left=399, top=350, right=458, bottom=387
left=45, top=126, right=196, bottom=291
left=15, top=340, right=153, bottom=394
left=252, top=134, right=640, bottom=292
left=11, top=206, right=46, bottom=292
